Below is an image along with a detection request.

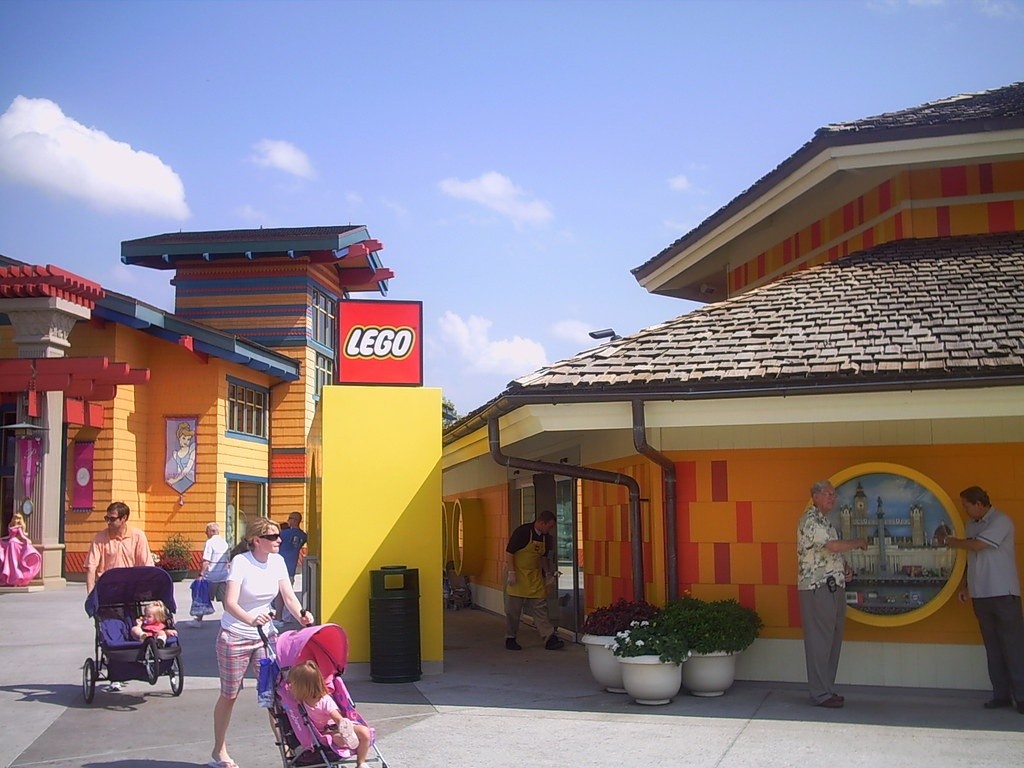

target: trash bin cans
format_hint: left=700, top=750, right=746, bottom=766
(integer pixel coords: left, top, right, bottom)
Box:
left=368, top=565, right=421, bottom=682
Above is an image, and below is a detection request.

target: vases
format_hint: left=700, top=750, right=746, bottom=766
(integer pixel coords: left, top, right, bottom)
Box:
left=616, top=650, right=692, bottom=706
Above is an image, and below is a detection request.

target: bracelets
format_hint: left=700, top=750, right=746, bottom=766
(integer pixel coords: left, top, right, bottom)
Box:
left=198, top=573, right=203, bottom=576
left=509, top=570, right=516, bottom=574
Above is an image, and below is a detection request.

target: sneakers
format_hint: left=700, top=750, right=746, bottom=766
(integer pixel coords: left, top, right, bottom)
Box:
left=819, top=695, right=844, bottom=707
left=334, top=718, right=359, bottom=750
left=506, top=638, right=522, bottom=650
left=110, top=681, right=128, bottom=691
left=273, top=619, right=284, bottom=628
left=186, top=618, right=202, bottom=628
left=545, top=635, right=564, bottom=650
left=358, top=763, right=369, bottom=768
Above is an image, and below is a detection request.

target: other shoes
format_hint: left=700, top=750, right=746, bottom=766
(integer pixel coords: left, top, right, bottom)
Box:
left=140, top=633, right=147, bottom=642
left=984, top=698, right=1013, bottom=708
left=1017, top=704, right=1024, bottom=714
left=157, top=637, right=165, bottom=649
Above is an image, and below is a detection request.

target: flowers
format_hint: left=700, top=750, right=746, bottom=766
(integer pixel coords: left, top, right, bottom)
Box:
left=603, top=618, right=690, bottom=665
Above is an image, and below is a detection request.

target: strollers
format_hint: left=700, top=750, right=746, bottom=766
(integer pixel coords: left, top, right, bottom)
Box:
left=257, top=609, right=389, bottom=768
left=82, top=566, right=185, bottom=704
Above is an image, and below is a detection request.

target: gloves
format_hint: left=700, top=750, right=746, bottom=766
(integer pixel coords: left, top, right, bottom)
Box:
left=508, top=571, right=516, bottom=586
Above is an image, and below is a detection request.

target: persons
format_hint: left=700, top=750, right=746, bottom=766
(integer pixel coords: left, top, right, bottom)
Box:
left=288, top=660, right=371, bottom=768
left=503, top=511, right=565, bottom=650
left=0, top=514, right=32, bottom=587
left=796, top=479, right=867, bottom=708
left=83, top=502, right=154, bottom=692
left=209, top=518, right=314, bottom=768
left=185, top=523, right=230, bottom=628
left=937, top=486, right=1024, bottom=715
left=272, top=512, right=307, bottom=629
left=131, top=601, right=177, bottom=649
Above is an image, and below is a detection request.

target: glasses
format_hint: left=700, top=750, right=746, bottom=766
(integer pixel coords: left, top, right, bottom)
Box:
left=289, top=517, right=294, bottom=520
left=104, top=515, right=120, bottom=522
left=259, top=534, right=280, bottom=541
left=818, top=492, right=839, bottom=500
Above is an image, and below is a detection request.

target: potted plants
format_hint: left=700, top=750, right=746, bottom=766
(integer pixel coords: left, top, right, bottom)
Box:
left=659, top=595, right=765, bottom=697
left=581, top=596, right=660, bottom=693
left=155, top=532, right=194, bottom=582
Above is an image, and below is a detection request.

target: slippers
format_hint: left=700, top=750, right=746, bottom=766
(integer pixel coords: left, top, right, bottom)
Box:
left=208, top=759, right=239, bottom=768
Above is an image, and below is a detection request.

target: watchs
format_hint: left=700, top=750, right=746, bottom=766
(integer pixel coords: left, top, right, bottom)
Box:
left=942, top=535, right=953, bottom=546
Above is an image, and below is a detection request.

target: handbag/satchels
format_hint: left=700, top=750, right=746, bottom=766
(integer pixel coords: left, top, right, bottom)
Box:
left=189, top=580, right=216, bottom=616
